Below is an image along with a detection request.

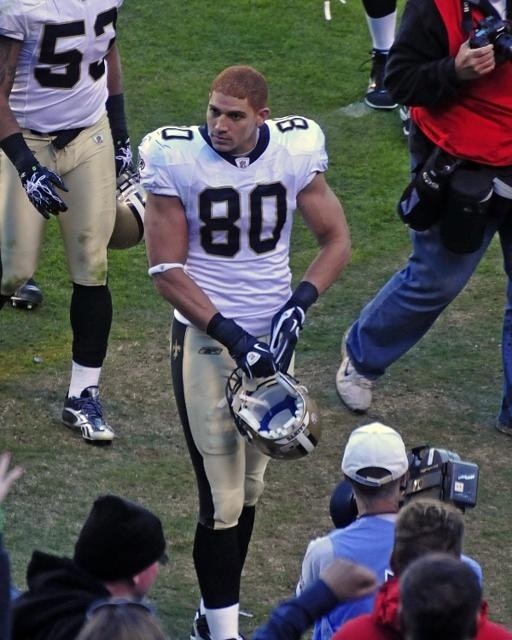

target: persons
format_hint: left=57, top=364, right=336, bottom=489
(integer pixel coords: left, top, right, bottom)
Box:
left=360, top=0, right=399, bottom=110
left=335, top=1, right=511, bottom=435
left=296, top=422, right=482, bottom=640
left=138, top=65, right=351, bottom=640
left=253, top=553, right=482, bottom=640
left=78, top=596, right=170, bottom=639
left=333, top=498, right=512, bottom=640
left=11, top=495, right=170, bottom=640
left=0, top=0, right=132, bottom=445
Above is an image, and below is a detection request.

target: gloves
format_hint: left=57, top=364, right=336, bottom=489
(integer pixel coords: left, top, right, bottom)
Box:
left=269, top=282, right=318, bottom=373
left=108, top=93, right=132, bottom=174
left=206, top=314, right=276, bottom=378
left=1, top=131, right=68, bottom=218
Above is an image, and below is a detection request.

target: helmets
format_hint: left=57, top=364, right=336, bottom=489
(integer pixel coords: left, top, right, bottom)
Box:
left=225, top=366, right=322, bottom=458
left=111, top=180, right=149, bottom=253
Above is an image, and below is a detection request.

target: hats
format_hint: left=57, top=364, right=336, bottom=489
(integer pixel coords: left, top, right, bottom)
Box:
left=77, top=494, right=167, bottom=579
left=342, top=422, right=409, bottom=486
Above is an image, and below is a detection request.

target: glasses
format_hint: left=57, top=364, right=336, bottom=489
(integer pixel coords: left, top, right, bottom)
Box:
left=158, top=554, right=169, bottom=566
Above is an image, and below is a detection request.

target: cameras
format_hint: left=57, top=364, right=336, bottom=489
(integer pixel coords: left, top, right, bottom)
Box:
left=469, top=14, right=512, bottom=63
left=328, top=446, right=478, bottom=528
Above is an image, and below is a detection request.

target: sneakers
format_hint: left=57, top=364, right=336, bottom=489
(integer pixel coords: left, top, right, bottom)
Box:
left=61, top=392, right=115, bottom=444
left=497, top=422, right=511, bottom=434
left=191, top=611, right=247, bottom=640
left=13, top=278, right=43, bottom=308
left=364, top=53, right=398, bottom=110
left=337, top=325, right=372, bottom=412
